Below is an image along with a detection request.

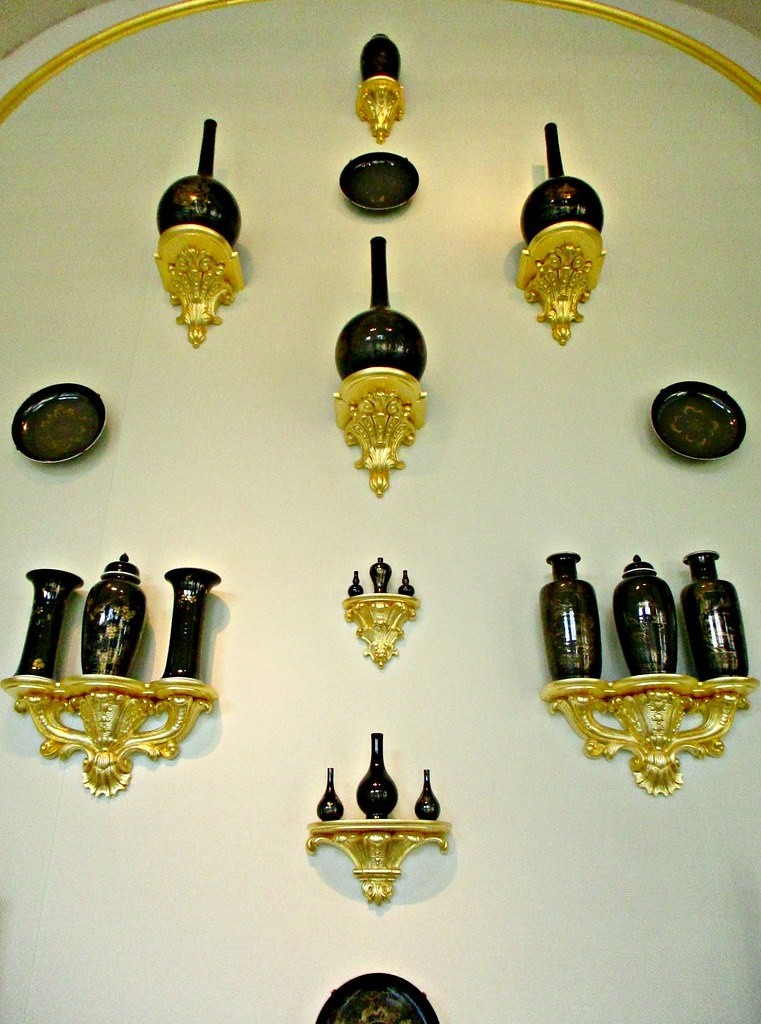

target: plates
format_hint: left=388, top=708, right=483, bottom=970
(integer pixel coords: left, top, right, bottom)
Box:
left=11, top=384, right=106, bottom=465
left=316, top=974, right=442, bottom=1024
left=339, top=151, right=420, bottom=209
left=650, top=381, right=746, bottom=460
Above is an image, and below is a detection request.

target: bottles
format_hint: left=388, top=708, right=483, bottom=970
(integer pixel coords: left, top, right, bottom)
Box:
left=415, top=769, right=440, bottom=820
left=156, top=118, right=240, bottom=250
left=346, top=558, right=415, bottom=597
left=517, top=123, right=604, bottom=244
left=538, top=550, right=750, bottom=680
left=360, top=31, right=400, bottom=82
left=335, top=236, right=427, bottom=386
left=355, top=733, right=398, bottom=820
left=317, top=767, right=343, bottom=821
left=81, top=553, right=147, bottom=675
